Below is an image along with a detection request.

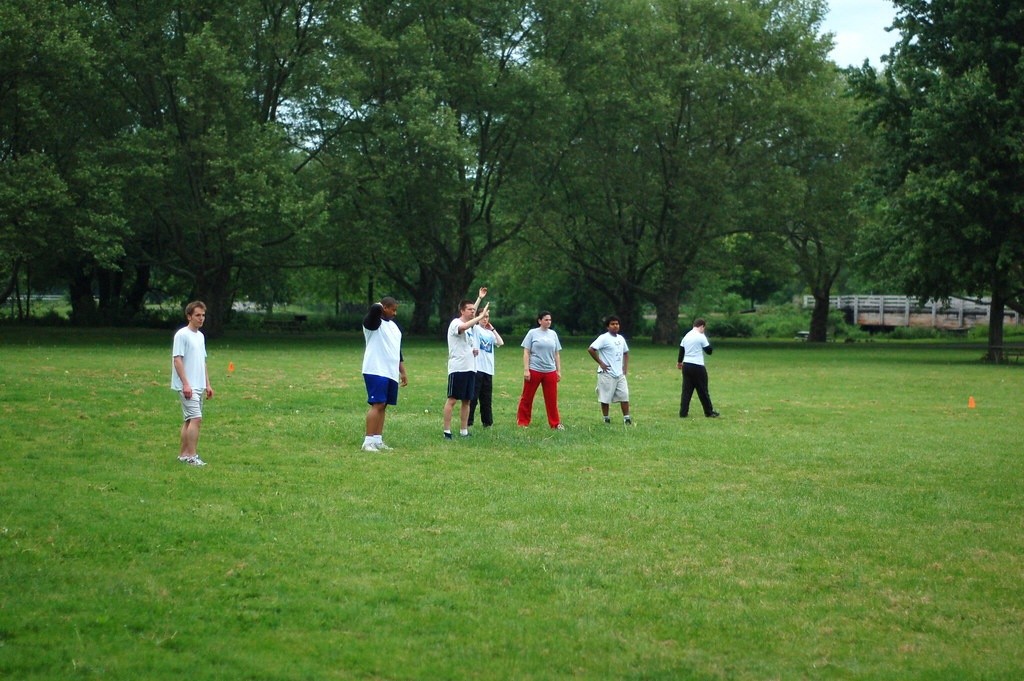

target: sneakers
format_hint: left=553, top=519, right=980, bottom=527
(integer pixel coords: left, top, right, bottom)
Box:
left=186, top=455, right=208, bottom=467
left=361, top=442, right=380, bottom=452
left=177, top=455, right=199, bottom=462
left=375, top=442, right=393, bottom=451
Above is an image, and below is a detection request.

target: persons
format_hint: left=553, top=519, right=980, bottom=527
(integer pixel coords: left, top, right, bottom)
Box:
left=171, top=301, right=213, bottom=465
left=362, top=297, right=408, bottom=451
left=676, top=318, right=720, bottom=418
left=517, top=311, right=562, bottom=428
left=588, top=315, right=631, bottom=425
left=467, top=287, right=504, bottom=427
left=443, top=300, right=490, bottom=439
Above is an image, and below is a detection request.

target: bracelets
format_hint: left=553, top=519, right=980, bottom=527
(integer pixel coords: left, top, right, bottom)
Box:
left=479, top=297, right=481, bottom=299
left=491, top=329, right=494, bottom=331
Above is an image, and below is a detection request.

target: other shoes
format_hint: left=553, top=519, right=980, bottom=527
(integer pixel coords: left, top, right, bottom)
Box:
left=602, top=418, right=610, bottom=423
left=556, top=423, right=564, bottom=430
left=459, top=432, right=472, bottom=437
left=444, top=432, right=453, bottom=440
left=624, top=416, right=631, bottom=426
left=709, top=411, right=720, bottom=417
